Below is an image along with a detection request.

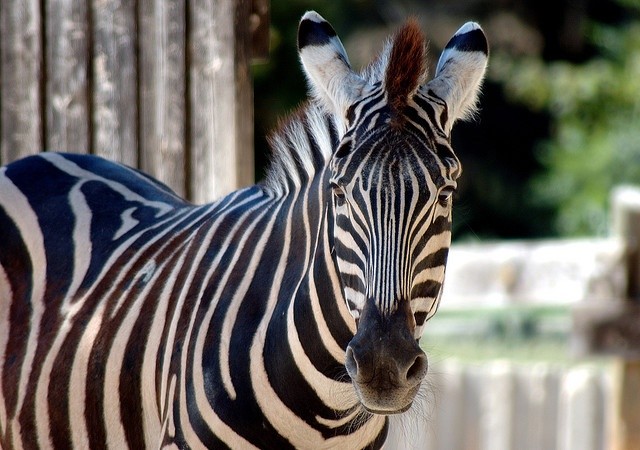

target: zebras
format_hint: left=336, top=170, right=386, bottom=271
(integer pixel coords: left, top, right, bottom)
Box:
left=0, top=11, right=490, bottom=450
